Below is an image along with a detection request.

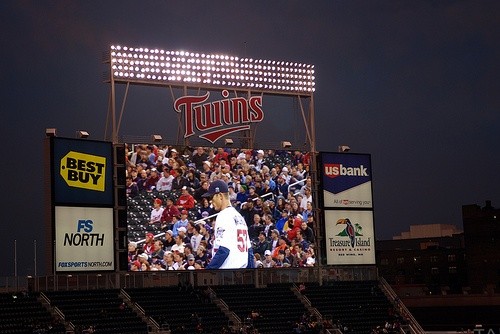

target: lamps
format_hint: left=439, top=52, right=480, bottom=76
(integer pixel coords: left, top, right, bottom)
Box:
left=280, top=141, right=291, bottom=150
left=152, top=134, right=162, bottom=143
left=338, top=145, right=350, bottom=153
left=224, top=138, right=234, bottom=145
left=46, top=127, right=57, bottom=136
left=79, top=131, right=89, bottom=138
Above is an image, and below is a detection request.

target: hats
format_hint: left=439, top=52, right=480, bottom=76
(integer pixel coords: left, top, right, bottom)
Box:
left=200, top=180, right=228, bottom=197
left=246, top=160, right=303, bottom=256
left=150, top=148, right=265, bottom=178
left=128, top=185, right=207, bottom=267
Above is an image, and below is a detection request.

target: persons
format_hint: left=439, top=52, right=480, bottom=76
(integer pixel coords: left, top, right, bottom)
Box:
left=125, top=142, right=315, bottom=271
left=300, top=312, right=317, bottom=321
left=100, top=308, right=108, bottom=318
left=220, top=325, right=259, bottom=334
left=196, top=322, right=203, bottom=333
left=338, top=320, right=345, bottom=329
left=201, top=180, right=255, bottom=269
left=119, top=300, right=125, bottom=312
left=33, top=318, right=60, bottom=334
left=177, top=280, right=193, bottom=292
left=300, top=282, right=306, bottom=295
left=372, top=320, right=400, bottom=334
left=247, top=308, right=261, bottom=320
